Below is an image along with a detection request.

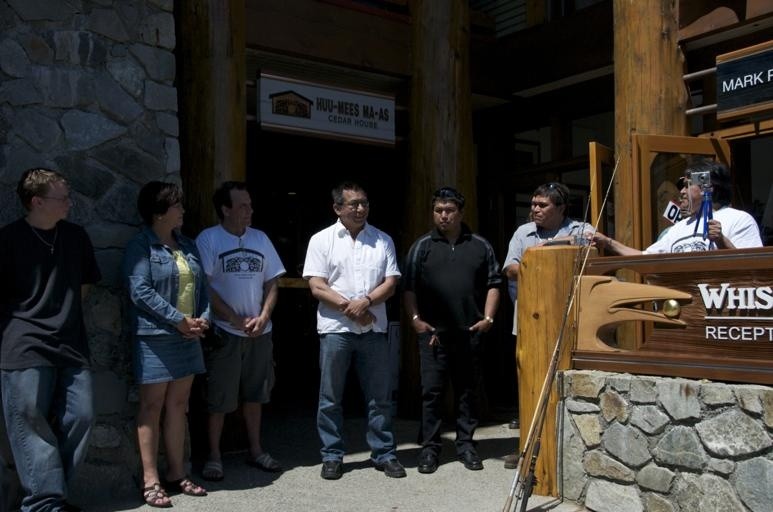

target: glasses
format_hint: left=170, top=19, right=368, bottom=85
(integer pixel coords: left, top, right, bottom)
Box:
left=39, top=191, right=72, bottom=201
left=546, top=182, right=567, bottom=204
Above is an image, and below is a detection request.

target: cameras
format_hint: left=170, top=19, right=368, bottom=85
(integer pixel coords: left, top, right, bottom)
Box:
left=689, top=171, right=711, bottom=188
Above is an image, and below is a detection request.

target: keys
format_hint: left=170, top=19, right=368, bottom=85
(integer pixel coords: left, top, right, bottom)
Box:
left=430, top=333, right=439, bottom=348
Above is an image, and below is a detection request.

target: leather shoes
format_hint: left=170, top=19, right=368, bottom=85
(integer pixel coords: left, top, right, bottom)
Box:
left=417, top=451, right=438, bottom=472
left=456, top=448, right=483, bottom=470
left=321, top=460, right=342, bottom=479
left=376, top=458, right=406, bottom=478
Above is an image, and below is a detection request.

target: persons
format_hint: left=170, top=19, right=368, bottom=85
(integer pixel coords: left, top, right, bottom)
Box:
left=192, top=180, right=286, bottom=481
left=123, top=180, right=212, bottom=507
left=402, top=189, right=502, bottom=473
left=501, top=183, right=598, bottom=469
left=0, top=169, right=100, bottom=512
left=588, top=159, right=763, bottom=257
left=300, top=183, right=406, bottom=480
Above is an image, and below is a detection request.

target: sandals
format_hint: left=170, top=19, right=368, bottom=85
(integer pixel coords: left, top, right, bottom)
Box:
left=141, top=483, right=171, bottom=507
left=160, top=475, right=206, bottom=497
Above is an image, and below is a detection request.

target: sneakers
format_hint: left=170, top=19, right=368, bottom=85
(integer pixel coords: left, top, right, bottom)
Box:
left=505, top=450, right=519, bottom=469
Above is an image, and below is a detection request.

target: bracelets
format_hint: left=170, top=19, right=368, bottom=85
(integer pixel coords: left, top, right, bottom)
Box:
left=608, top=239, right=613, bottom=247
left=485, top=315, right=494, bottom=326
left=408, top=312, right=420, bottom=322
left=366, top=295, right=371, bottom=305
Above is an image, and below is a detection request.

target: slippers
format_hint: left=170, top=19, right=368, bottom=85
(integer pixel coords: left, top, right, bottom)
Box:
left=245, top=452, right=281, bottom=472
left=201, top=461, right=225, bottom=482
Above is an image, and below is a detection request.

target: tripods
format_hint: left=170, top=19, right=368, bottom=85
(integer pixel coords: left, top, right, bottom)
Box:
left=692, top=188, right=718, bottom=241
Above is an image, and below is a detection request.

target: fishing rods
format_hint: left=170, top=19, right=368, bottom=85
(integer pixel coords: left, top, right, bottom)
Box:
left=503, top=154, right=622, bottom=512
left=520, top=163, right=599, bottom=512
